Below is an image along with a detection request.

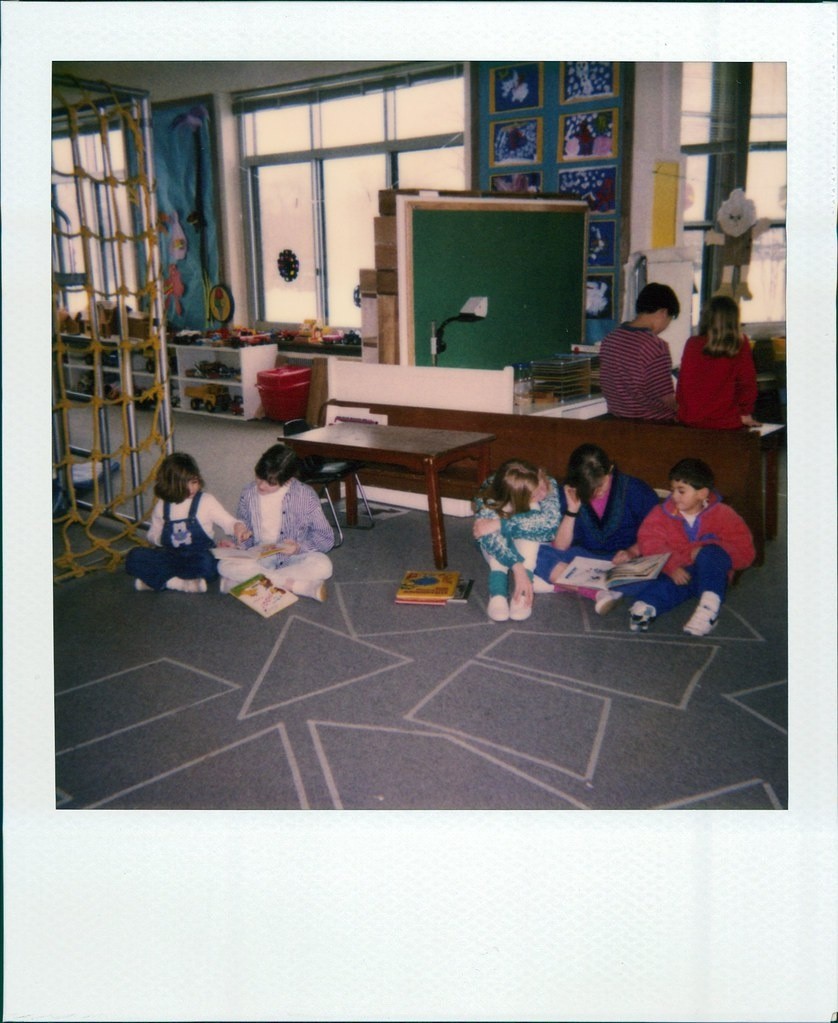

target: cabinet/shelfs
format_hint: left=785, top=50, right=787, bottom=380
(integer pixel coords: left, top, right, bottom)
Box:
left=58, top=332, right=277, bottom=422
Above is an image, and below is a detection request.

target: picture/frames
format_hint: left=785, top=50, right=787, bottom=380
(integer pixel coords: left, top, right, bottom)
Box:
left=488, top=61, right=620, bottom=319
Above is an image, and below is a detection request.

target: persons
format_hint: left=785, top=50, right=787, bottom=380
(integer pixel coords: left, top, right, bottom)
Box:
left=534, top=444, right=660, bottom=615
left=599, top=283, right=679, bottom=421
left=126, top=452, right=252, bottom=593
left=630, top=458, right=756, bottom=637
left=675, top=296, right=762, bottom=427
left=472, top=459, right=562, bottom=621
left=217, top=444, right=335, bottom=602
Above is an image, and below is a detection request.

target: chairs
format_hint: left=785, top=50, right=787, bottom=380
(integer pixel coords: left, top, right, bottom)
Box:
left=282, top=420, right=375, bottom=549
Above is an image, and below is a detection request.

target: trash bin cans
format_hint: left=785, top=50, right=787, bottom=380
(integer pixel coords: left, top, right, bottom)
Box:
left=257, top=365, right=311, bottom=420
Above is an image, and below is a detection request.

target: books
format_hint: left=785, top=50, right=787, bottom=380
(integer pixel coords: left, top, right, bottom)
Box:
left=395, top=570, right=474, bottom=605
left=209, top=544, right=288, bottom=560
left=554, top=552, right=672, bottom=590
left=231, top=574, right=298, bottom=620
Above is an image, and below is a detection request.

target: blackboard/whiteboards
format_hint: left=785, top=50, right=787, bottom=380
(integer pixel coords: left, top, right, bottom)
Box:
left=398, top=195, right=591, bottom=406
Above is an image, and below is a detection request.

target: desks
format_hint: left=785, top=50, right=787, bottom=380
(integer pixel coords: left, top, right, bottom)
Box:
left=275, top=422, right=495, bottom=570
left=589, top=411, right=787, bottom=538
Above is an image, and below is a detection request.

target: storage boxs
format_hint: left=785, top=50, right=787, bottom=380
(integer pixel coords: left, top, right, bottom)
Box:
left=254, top=365, right=312, bottom=421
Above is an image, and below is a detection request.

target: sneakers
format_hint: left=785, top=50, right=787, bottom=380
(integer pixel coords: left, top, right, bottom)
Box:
left=683, top=603, right=719, bottom=636
left=629, top=604, right=656, bottom=630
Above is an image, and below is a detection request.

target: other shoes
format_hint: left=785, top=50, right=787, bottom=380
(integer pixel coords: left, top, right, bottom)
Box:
left=292, top=578, right=327, bottom=602
left=183, top=578, right=206, bottom=593
left=510, top=595, right=531, bottom=620
left=487, top=595, right=509, bottom=621
left=135, top=579, right=153, bottom=591
left=594, top=590, right=623, bottom=616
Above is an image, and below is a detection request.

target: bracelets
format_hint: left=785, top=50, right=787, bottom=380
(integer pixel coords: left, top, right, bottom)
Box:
left=565, top=510, right=577, bottom=517
